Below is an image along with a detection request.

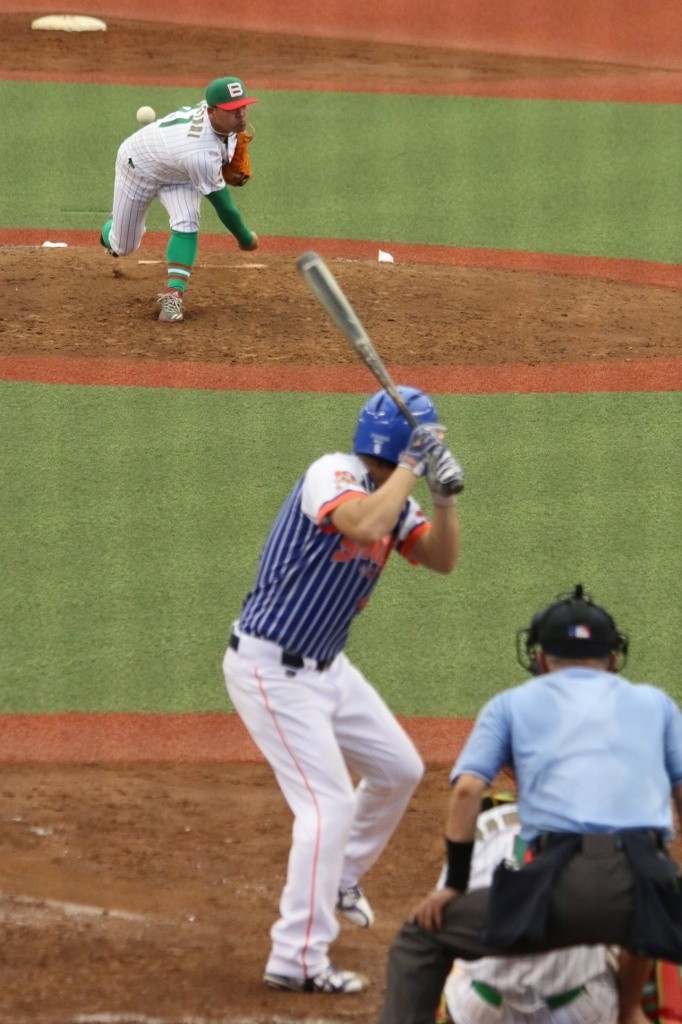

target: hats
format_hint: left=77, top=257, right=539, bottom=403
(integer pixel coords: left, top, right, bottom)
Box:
left=535, top=600, right=614, bottom=657
left=206, top=77, right=259, bottom=110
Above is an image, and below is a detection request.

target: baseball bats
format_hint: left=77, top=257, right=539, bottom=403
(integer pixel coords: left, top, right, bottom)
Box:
left=297, top=253, right=464, bottom=496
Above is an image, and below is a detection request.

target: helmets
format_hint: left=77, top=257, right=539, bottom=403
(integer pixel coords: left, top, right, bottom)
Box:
left=353, top=386, right=439, bottom=464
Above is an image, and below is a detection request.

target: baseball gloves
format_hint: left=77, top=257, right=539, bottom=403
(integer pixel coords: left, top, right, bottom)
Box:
left=222, top=130, right=253, bottom=186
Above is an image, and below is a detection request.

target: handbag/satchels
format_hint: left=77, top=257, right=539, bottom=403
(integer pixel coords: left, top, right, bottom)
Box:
left=620, top=826, right=682, bottom=967
left=477, top=831, right=581, bottom=953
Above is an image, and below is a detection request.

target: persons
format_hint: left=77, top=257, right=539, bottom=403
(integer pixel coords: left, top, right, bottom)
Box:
left=381, top=601, right=682, bottom=1024
left=100, top=77, right=259, bottom=322
left=222, top=386, right=466, bottom=994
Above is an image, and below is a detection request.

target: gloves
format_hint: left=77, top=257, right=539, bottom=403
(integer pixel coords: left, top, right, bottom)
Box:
left=426, top=446, right=464, bottom=507
left=397, top=423, right=448, bottom=477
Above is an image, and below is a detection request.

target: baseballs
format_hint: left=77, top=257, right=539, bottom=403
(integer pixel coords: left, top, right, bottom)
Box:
left=137, top=106, right=155, bottom=124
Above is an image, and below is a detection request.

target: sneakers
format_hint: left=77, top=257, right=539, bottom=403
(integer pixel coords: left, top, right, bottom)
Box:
left=337, top=883, right=374, bottom=928
left=263, top=966, right=369, bottom=994
left=157, top=288, right=184, bottom=323
left=107, top=248, right=119, bottom=258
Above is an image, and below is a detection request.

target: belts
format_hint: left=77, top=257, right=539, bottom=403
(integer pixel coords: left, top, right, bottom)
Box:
left=230, top=625, right=333, bottom=673
left=471, top=982, right=586, bottom=1011
